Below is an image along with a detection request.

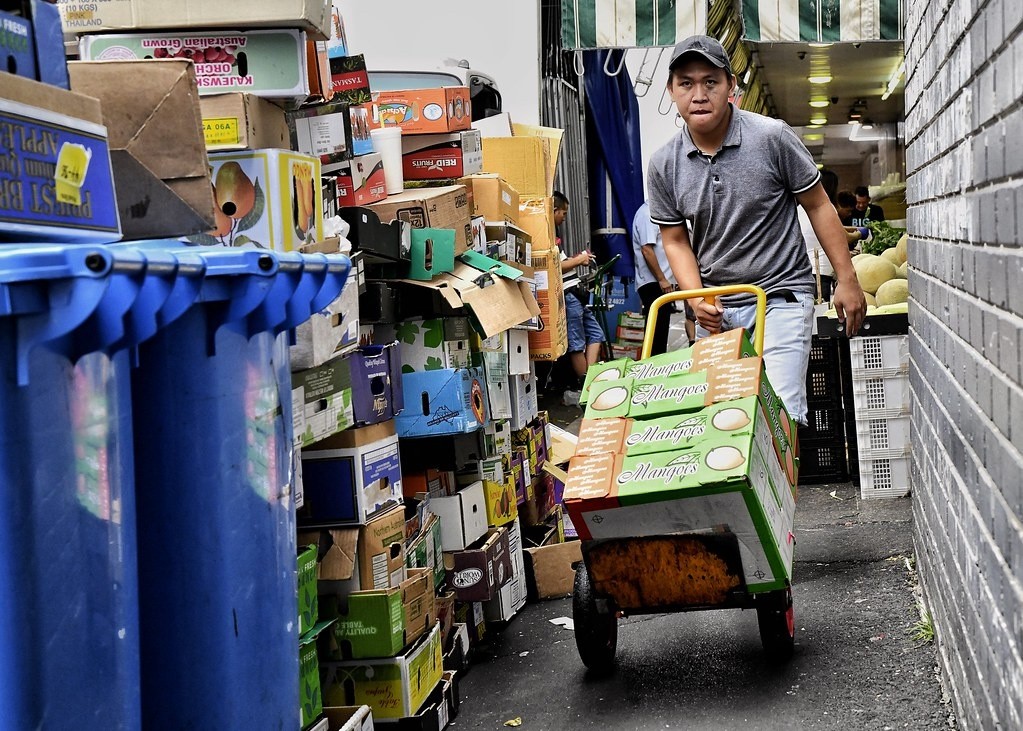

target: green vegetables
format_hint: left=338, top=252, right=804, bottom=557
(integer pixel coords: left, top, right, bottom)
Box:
left=860, top=219, right=907, bottom=255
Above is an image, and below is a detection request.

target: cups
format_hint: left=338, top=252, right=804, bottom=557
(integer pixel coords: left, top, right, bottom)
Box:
left=369, top=126, right=404, bottom=195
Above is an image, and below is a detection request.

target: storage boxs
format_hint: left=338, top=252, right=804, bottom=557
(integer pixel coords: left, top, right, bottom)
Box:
left=0, top=0, right=911, bottom=731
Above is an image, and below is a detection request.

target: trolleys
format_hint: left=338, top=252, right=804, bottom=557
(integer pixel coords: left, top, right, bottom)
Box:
left=570, top=281, right=794, bottom=669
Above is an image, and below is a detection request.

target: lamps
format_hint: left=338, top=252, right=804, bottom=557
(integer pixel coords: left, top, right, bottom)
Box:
left=861, top=120, right=874, bottom=129
left=881, top=61, right=905, bottom=100
left=847, top=116, right=859, bottom=125
left=853, top=100, right=868, bottom=110
left=849, top=109, right=863, bottom=117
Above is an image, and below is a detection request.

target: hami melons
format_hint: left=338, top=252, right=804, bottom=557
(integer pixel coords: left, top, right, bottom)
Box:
left=831, top=231, right=909, bottom=307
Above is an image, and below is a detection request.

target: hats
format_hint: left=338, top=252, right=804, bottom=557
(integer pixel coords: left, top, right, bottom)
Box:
left=668, top=35, right=733, bottom=79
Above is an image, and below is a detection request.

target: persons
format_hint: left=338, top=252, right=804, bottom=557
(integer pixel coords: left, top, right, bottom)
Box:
left=819, top=167, right=841, bottom=205
left=648, top=36, right=868, bottom=427
left=632, top=202, right=677, bottom=354
left=854, top=186, right=886, bottom=220
left=683, top=300, right=696, bottom=345
left=795, top=203, right=869, bottom=301
left=838, top=191, right=859, bottom=217
left=554, top=191, right=608, bottom=384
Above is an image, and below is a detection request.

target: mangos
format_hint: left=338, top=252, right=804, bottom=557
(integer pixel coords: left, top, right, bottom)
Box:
left=207, top=161, right=256, bottom=237
